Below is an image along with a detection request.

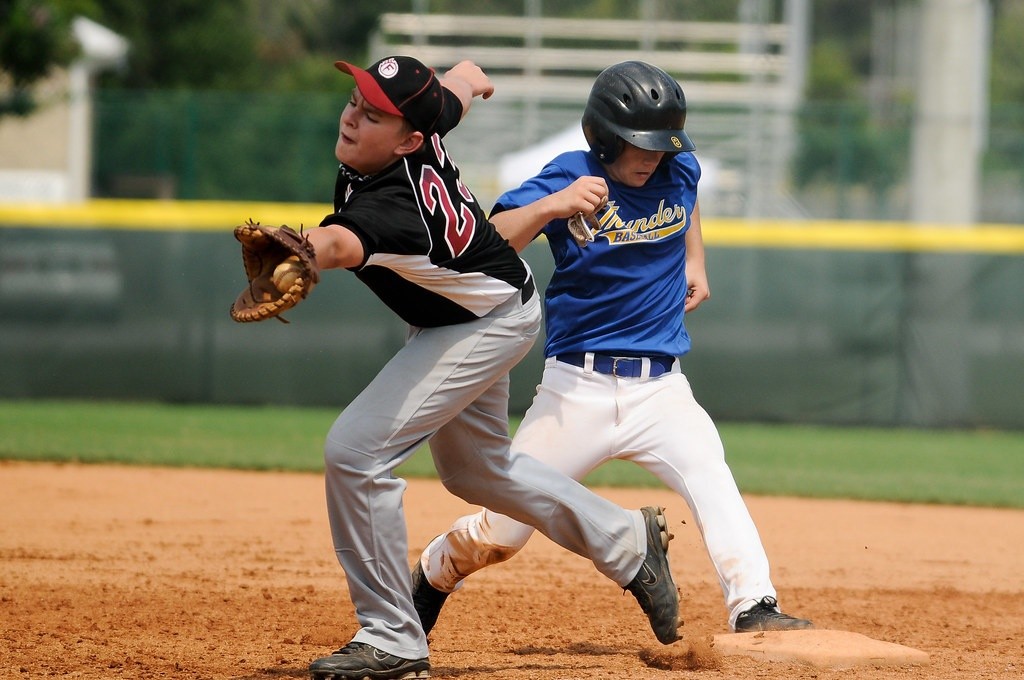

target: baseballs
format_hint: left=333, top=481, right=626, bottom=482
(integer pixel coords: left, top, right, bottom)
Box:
left=271, top=260, right=305, bottom=295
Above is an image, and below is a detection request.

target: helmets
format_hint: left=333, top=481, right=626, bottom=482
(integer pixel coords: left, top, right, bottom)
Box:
left=582, top=61, right=697, bottom=165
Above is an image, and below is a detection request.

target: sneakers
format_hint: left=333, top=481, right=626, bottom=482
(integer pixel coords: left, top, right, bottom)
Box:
left=735, top=596, right=813, bottom=631
left=308, top=641, right=431, bottom=680
left=410, top=558, right=449, bottom=636
left=621, top=505, right=685, bottom=645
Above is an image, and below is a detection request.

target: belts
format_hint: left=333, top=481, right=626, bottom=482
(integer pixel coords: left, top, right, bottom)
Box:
left=556, top=351, right=672, bottom=379
left=521, top=276, right=535, bottom=306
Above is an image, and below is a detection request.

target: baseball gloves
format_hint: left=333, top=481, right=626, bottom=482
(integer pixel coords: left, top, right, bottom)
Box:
left=223, top=220, right=324, bottom=323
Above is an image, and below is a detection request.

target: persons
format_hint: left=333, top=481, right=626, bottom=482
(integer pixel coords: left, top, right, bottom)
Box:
left=230, top=56, right=684, bottom=679
left=408, top=61, right=814, bottom=636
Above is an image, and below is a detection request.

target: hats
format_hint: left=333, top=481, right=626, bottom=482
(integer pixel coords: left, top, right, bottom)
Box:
left=335, top=56, right=444, bottom=142
